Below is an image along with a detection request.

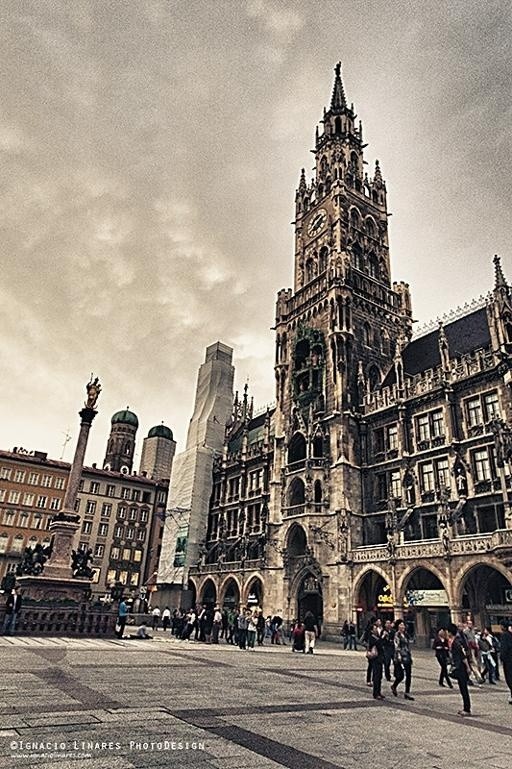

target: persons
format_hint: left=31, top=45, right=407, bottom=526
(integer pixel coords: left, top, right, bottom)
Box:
left=152, top=604, right=319, bottom=655
left=136, top=621, right=147, bottom=638
left=1, top=587, right=21, bottom=634
left=434, top=621, right=511, bottom=717
left=340, top=617, right=415, bottom=703
left=117, top=597, right=128, bottom=638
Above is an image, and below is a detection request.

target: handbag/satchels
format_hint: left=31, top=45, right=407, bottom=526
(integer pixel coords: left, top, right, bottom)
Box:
left=394, top=656, right=411, bottom=664
left=449, top=666, right=469, bottom=681
left=366, top=646, right=378, bottom=659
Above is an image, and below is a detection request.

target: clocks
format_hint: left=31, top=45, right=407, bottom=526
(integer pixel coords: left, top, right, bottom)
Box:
left=307, top=208, right=328, bottom=238
